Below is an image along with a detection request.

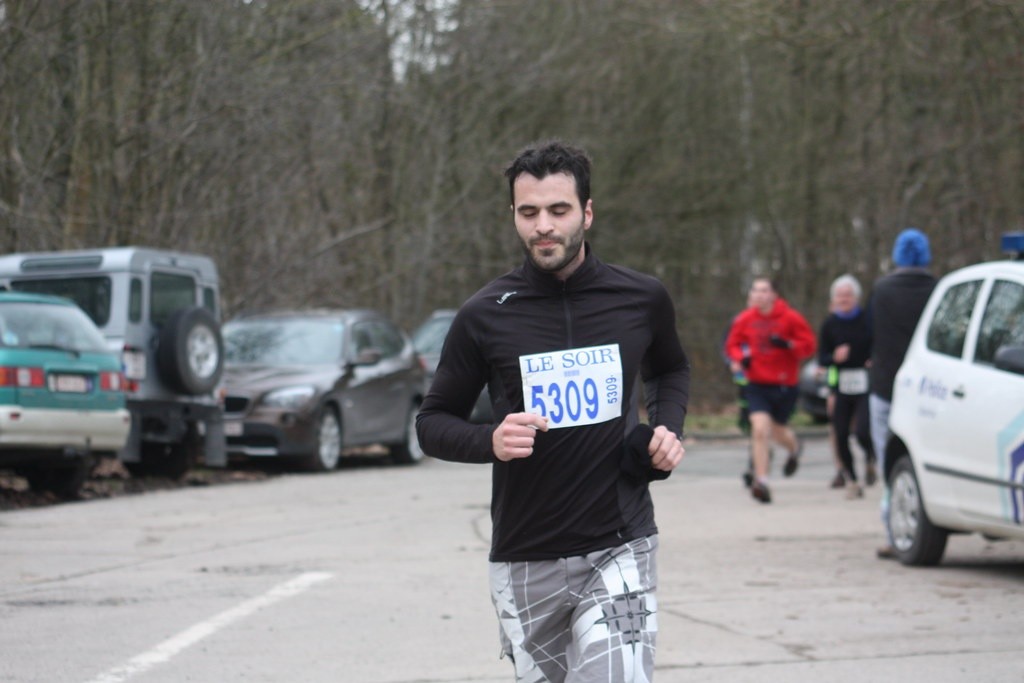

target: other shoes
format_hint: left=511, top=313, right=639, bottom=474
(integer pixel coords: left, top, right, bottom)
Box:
left=831, top=472, right=856, bottom=488
left=864, top=455, right=878, bottom=485
left=847, top=485, right=862, bottom=500
left=877, top=545, right=895, bottom=559
left=753, top=485, right=771, bottom=504
left=743, top=471, right=752, bottom=488
left=784, top=443, right=802, bottom=476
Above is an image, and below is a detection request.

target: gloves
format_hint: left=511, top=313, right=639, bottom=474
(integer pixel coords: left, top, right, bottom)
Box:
left=731, top=371, right=749, bottom=388
left=770, top=335, right=789, bottom=350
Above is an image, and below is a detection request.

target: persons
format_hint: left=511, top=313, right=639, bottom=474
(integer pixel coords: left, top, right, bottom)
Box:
left=723, top=276, right=818, bottom=503
left=817, top=230, right=939, bottom=500
left=415, top=146, right=689, bottom=683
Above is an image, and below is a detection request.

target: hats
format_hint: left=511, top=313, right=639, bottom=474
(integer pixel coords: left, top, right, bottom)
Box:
left=892, top=227, right=931, bottom=267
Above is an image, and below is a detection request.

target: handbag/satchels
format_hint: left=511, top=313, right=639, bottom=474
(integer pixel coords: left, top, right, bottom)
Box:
left=837, top=369, right=868, bottom=395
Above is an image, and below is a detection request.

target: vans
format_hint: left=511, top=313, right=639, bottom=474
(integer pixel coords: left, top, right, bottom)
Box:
left=0, top=246, right=228, bottom=479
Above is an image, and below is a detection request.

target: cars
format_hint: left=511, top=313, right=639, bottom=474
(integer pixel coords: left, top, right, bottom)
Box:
left=197, top=305, right=428, bottom=473
left=0, top=292, right=134, bottom=500
left=410, top=308, right=494, bottom=424
left=883, top=233, right=1023, bottom=567
